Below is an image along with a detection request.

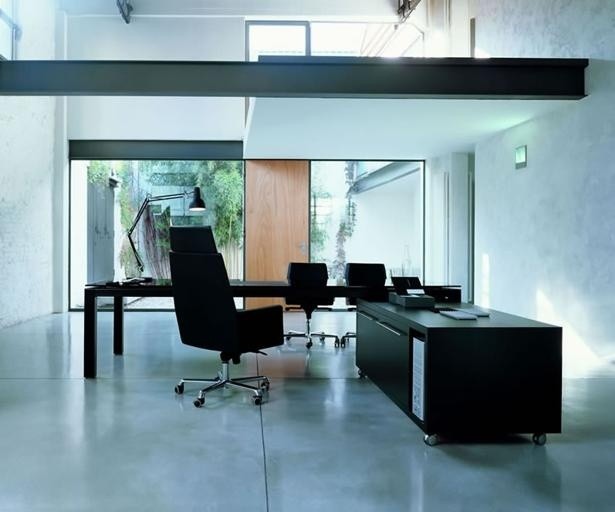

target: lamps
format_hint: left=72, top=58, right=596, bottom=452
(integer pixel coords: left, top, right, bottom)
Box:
left=123, top=187, right=206, bottom=282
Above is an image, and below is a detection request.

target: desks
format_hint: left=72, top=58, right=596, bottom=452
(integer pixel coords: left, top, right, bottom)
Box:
left=354, top=298, right=563, bottom=447
left=82, top=277, right=462, bottom=380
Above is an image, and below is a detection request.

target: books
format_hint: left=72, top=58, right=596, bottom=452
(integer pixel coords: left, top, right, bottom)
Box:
left=454, top=304, right=490, bottom=317
left=439, top=308, right=477, bottom=319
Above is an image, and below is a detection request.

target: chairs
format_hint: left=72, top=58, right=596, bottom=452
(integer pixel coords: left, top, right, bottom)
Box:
left=284, top=261, right=339, bottom=349
left=168, top=227, right=284, bottom=405
left=340, top=263, right=388, bottom=347
